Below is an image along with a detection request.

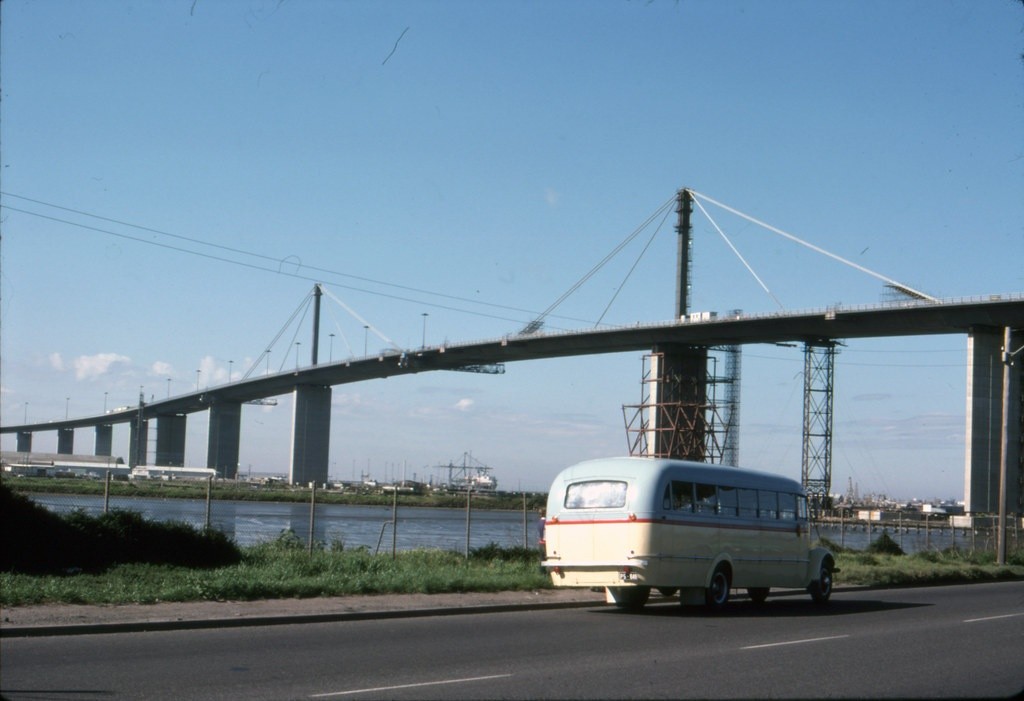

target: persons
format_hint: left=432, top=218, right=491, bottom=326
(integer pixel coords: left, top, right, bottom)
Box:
left=537, top=509, right=549, bottom=575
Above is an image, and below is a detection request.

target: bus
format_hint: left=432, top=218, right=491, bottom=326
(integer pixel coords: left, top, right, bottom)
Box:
left=543, top=456, right=843, bottom=615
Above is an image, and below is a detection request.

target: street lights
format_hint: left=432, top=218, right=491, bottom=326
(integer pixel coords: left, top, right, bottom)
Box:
left=364, top=326, right=369, bottom=359
left=421, top=313, right=429, bottom=349
left=329, top=333, right=334, bottom=363
left=66, top=397, right=69, bottom=422
left=265, top=349, right=270, bottom=375
left=229, top=360, right=233, bottom=384
left=25, top=402, right=29, bottom=425
left=195, top=370, right=200, bottom=392
left=103, top=392, right=108, bottom=413
left=295, top=342, right=301, bottom=371
left=167, top=378, right=171, bottom=401
